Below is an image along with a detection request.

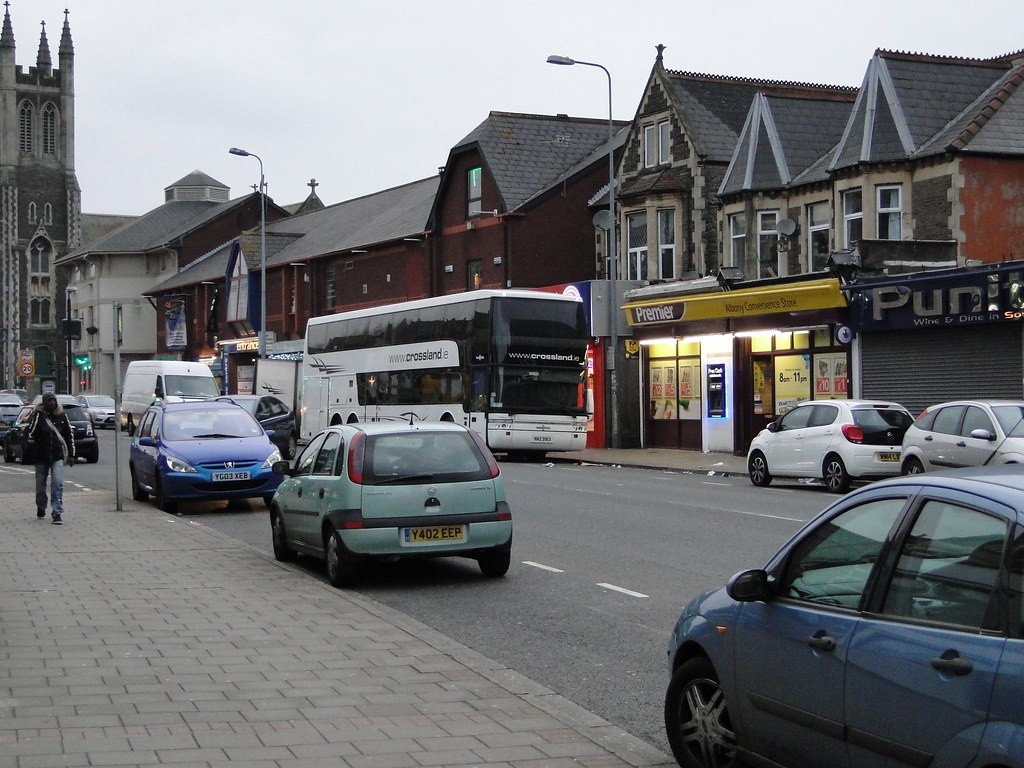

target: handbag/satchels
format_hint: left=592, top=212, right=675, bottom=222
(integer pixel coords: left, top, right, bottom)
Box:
left=61, top=438, right=69, bottom=466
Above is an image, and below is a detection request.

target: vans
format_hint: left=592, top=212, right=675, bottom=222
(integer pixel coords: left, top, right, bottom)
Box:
left=121, top=360, right=221, bottom=437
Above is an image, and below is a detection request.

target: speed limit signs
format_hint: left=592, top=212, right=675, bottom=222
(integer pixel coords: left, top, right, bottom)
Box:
left=21, top=363, right=33, bottom=375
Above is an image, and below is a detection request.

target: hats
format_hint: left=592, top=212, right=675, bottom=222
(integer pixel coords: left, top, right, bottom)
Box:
left=42, top=392, right=57, bottom=411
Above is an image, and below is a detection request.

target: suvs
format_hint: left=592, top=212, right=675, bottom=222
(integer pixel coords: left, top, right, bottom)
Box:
left=129, top=397, right=284, bottom=514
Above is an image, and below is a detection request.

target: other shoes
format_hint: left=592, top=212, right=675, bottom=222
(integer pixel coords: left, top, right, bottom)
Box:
left=52, top=514, right=62, bottom=525
left=37, top=507, right=45, bottom=517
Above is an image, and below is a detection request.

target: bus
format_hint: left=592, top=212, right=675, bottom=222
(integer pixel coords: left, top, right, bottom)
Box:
left=300, top=290, right=589, bottom=460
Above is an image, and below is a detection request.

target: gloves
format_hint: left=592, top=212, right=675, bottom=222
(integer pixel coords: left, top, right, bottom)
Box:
left=69, top=456, right=75, bottom=466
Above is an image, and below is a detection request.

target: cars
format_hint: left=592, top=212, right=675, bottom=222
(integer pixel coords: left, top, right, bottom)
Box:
left=181, top=394, right=297, bottom=460
left=664, top=464, right=1023, bottom=768
left=33, top=395, right=91, bottom=422
left=270, top=420, right=513, bottom=588
left=75, top=395, right=122, bottom=428
left=746, top=400, right=916, bottom=494
left=900, top=399, right=1024, bottom=476
left=2, top=403, right=99, bottom=464
left=0, top=393, right=24, bottom=445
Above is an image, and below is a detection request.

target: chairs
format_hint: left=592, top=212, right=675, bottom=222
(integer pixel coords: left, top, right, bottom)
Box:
left=210, top=414, right=236, bottom=435
left=251, top=401, right=264, bottom=421
left=165, top=422, right=184, bottom=440
left=958, top=539, right=1011, bottom=632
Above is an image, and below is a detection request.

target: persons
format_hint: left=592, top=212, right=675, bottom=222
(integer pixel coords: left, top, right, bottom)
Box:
left=27, top=391, right=76, bottom=524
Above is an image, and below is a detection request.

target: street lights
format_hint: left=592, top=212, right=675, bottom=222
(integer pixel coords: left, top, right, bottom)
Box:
left=229, top=147, right=266, bottom=359
left=67, top=287, right=77, bottom=394
left=546, top=55, right=621, bottom=448
